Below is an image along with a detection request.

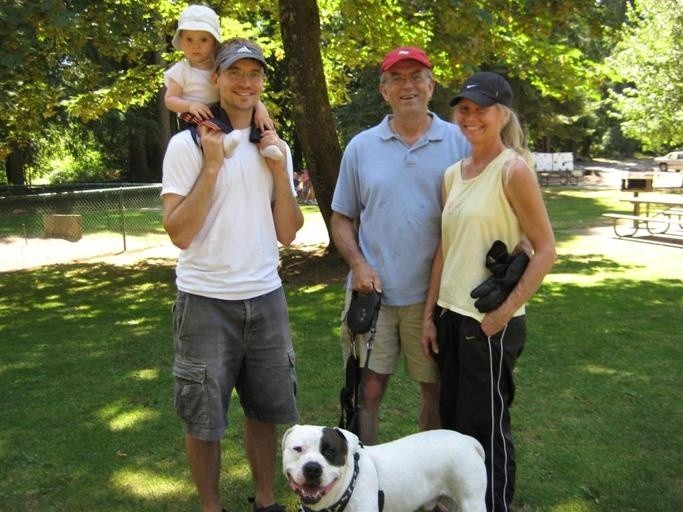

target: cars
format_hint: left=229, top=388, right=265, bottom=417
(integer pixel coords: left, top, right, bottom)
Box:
left=653, top=151, right=683, bottom=172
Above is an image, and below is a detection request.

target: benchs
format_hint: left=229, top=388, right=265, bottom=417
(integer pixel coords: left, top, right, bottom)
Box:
left=601, top=207, right=683, bottom=238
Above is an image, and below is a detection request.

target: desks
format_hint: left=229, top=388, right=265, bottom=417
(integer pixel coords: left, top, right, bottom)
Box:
left=619, top=193, right=683, bottom=238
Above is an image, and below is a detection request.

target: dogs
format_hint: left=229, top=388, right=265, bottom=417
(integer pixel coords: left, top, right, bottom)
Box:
left=281, top=423, right=488, bottom=512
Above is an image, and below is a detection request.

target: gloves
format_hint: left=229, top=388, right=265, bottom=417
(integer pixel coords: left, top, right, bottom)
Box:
left=470, top=240, right=530, bottom=313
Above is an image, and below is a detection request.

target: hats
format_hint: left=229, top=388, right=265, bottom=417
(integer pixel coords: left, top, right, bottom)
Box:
left=449, top=72, right=513, bottom=107
left=171, top=5, right=221, bottom=50
left=215, top=44, right=267, bottom=73
left=379, top=47, right=432, bottom=73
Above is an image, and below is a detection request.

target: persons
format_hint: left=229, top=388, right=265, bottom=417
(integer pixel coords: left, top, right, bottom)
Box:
left=293, top=167, right=318, bottom=206
left=165, top=4, right=283, bottom=161
left=331, top=45, right=473, bottom=448
left=418, top=71, right=557, bottom=512
left=159, top=39, right=303, bottom=512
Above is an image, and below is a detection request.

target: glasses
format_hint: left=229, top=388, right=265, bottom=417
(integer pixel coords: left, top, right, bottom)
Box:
left=220, top=70, right=263, bottom=79
